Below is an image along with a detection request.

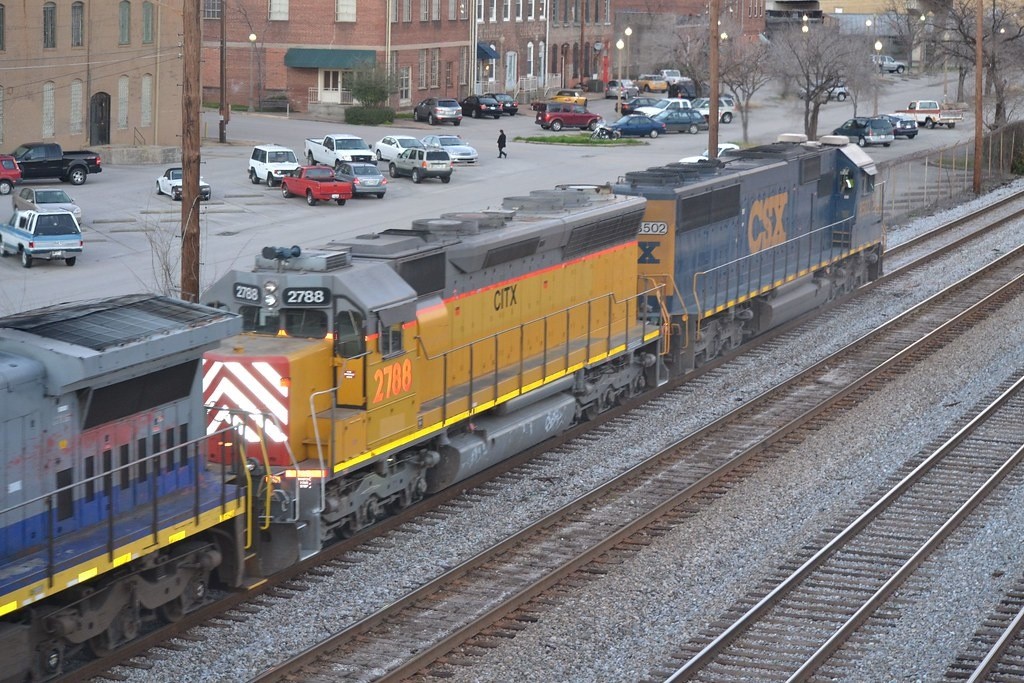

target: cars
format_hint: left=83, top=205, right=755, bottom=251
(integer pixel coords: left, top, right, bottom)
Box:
left=865, top=115, right=919, bottom=139
left=413, top=97, right=462, bottom=126
left=11, top=186, right=82, bottom=229
left=418, top=134, right=478, bottom=168
left=155, top=167, right=212, bottom=201
left=334, top=162, right=388, bottom=199
left=459, top=66, right=739, bottom=139
left=374, top=136, right=425, bottom=162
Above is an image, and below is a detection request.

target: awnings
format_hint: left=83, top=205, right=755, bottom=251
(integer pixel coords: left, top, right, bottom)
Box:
left=283, top=48, right=376, bottom=69
left=479, top=43, right=501, bottom=59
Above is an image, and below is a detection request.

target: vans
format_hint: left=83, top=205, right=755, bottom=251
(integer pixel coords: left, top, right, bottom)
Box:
left=248, top=144, right=301, bottom=188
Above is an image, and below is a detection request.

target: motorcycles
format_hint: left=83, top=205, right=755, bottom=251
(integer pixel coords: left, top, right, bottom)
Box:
left=589, top=125, right=618, bottom=141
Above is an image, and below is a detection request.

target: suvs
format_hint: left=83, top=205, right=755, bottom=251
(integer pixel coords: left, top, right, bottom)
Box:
left=388, top=145, right=452, bottom=184
left=799, top=77, right=851, bottom=103
left=1, top=153, right=24, bottom=196
left=865, top=54, right=908, bottom=75
left=831, top=115, right=895, bottom=148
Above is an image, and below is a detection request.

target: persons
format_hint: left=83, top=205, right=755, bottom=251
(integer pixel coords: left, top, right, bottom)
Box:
left=496, top=129, right=507, bottom=159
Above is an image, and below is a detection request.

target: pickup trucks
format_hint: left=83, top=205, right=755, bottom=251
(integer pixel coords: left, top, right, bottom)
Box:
left=281, top=166, right=353, bottom=207
left=4, top=143, right=102, bottom=187
left=1, top=209, right=84, bottom=270
left=536, top=102, right=602, bottom=132
left=304, top=134, right=378, bottom=172
left=893, top=100, right=964, bottom=130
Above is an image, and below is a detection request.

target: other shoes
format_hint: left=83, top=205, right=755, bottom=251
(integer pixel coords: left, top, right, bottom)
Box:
left=497, top=156, right=501, bottom=158
left=505, top=154, right=507, bottom=158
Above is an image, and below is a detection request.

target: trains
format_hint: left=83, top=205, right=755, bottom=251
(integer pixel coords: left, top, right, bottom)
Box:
left=0, top=134, right=886, bottom=683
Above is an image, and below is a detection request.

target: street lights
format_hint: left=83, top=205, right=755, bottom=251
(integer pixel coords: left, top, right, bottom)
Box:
left=863, top=12, right=950, bottom=119
left=614, top=25, right=633, bottom=123
